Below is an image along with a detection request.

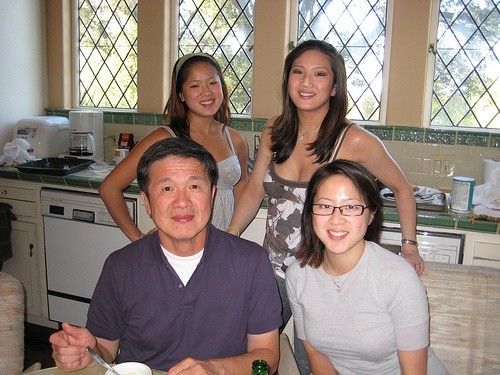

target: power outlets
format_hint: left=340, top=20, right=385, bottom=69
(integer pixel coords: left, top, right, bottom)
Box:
left=430, top=154, right=457, bottom=180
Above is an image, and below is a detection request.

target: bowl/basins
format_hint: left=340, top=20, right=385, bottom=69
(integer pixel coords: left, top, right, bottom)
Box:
left=105, top=362, right=152, bottom=375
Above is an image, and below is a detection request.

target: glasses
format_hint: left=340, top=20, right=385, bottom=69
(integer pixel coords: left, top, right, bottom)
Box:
left=310, top=203, right=370, bottom=217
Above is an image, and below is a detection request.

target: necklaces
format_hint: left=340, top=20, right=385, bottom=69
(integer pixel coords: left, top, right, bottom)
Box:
left=299, top=120, right=320, bottom=141
left=325, top=244, right=364, bottom=293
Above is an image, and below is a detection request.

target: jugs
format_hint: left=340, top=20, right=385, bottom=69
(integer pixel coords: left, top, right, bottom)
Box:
left=69, top=133, right=93, bottom=156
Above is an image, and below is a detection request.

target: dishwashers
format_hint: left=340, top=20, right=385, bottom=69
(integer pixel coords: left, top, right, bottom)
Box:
left=377, top=223, right=466, bottom=266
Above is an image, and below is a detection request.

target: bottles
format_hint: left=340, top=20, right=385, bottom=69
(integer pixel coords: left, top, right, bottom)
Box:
left=251, top=359, right=268, bottom=375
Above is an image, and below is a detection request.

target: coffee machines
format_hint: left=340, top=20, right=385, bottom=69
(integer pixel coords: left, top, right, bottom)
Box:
left=69, top=110, right=104, bottom=163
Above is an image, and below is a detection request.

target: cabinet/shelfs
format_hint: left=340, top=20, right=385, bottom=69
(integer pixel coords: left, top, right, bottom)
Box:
left=238, top=208, right=500, bottom=352
left=0, top=177, right=60, bottom=332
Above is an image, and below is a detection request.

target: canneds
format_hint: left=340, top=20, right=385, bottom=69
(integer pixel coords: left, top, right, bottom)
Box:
left=450, top=176, right=475, bottom=214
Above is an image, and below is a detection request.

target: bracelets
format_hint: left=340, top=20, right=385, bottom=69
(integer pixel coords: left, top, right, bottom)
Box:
left=402, top=239, right=417, bottom=246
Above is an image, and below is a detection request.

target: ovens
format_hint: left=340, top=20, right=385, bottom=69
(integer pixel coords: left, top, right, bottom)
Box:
left=40, top=188, right=136, bottom=328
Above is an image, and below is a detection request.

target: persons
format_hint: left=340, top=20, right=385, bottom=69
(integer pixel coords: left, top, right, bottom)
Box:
left=98, top=52, right=249, bottom=242
left=285, top=159, right=429, bottom=375
left=227, top=39, right=425, bottom=375
left=50, top=136, right=284, bottom=375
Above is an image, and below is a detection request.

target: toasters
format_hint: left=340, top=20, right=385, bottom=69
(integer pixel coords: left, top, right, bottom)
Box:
left=13, top=116, right=69, bottom=159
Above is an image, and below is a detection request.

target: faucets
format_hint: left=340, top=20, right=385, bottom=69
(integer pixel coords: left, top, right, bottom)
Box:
left=254, top=145, right=260, bottom=160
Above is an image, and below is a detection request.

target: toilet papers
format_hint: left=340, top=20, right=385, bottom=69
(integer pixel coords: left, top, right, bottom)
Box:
left=482, top=158, right=500, bottom=183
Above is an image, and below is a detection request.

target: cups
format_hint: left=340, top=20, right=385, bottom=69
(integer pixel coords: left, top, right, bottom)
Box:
left=114, top=149, right=129, bottom=166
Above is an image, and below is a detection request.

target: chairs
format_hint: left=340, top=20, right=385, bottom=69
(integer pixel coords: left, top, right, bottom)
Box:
left=417, top=261, right=500, bottom=375
left=274, top=314, right=450, bottom=375
